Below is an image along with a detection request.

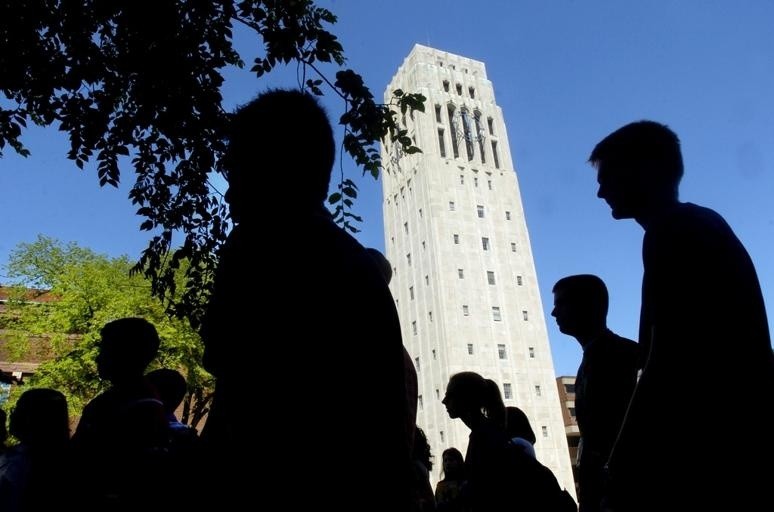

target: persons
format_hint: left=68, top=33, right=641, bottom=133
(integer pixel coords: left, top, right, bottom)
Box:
left=181, top=86, right=403, bottom=508
left=0, top=315, right=204, bottom=511
left=578, top=120, right=772, bottom=511
left=549, top=275, right=639, bottom=510
left=405, top=371, right=579, bottom=511
